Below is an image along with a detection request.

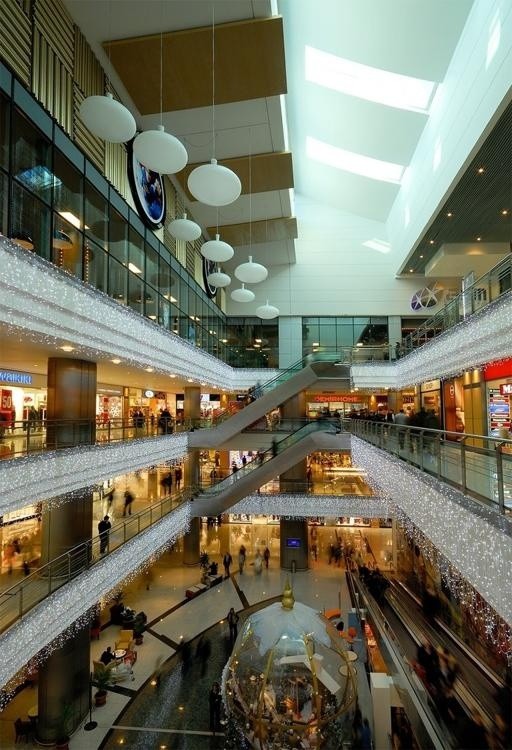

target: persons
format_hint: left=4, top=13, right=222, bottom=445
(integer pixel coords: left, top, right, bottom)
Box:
left=379, top=339, right=392, bottom=361
left=393, top=341, right=401, bottom=361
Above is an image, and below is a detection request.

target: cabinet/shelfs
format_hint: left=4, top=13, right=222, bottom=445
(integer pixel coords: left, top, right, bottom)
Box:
left=490, top=387, right=510, bottom=436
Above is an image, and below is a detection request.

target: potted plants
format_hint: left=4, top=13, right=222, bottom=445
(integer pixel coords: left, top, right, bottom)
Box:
left=91, top=666, right=111, bottom=707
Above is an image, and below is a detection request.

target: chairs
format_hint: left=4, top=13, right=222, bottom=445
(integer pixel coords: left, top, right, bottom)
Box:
left=14, top=717, right=30, bottom=745
left=93, top=603, right=147, bottom=681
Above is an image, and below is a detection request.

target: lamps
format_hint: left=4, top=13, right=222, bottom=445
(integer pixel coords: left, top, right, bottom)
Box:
left=168, top=165, right=202, bottom=242
left=201, top=142, right=280, bottom=320
left=187, top=1, right=242, bottom=208
left=80, top=0, right=136, bottom=143
left=132, top=0, right=189, bottom=174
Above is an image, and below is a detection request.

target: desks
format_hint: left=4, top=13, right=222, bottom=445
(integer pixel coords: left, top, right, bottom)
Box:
left=27, top=704, right=39, bottom=728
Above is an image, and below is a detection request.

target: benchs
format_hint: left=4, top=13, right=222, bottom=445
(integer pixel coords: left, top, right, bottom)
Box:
left=186, top=573, right=222, bottom=598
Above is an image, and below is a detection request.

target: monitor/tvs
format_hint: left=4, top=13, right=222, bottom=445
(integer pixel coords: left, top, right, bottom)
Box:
left=286, top=537, right=301, bottom=549
left=236, top=394, right=246, bottom=401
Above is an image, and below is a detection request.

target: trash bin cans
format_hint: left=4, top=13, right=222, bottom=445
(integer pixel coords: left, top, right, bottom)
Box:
left=291, top=560, right=296, bottom=573
